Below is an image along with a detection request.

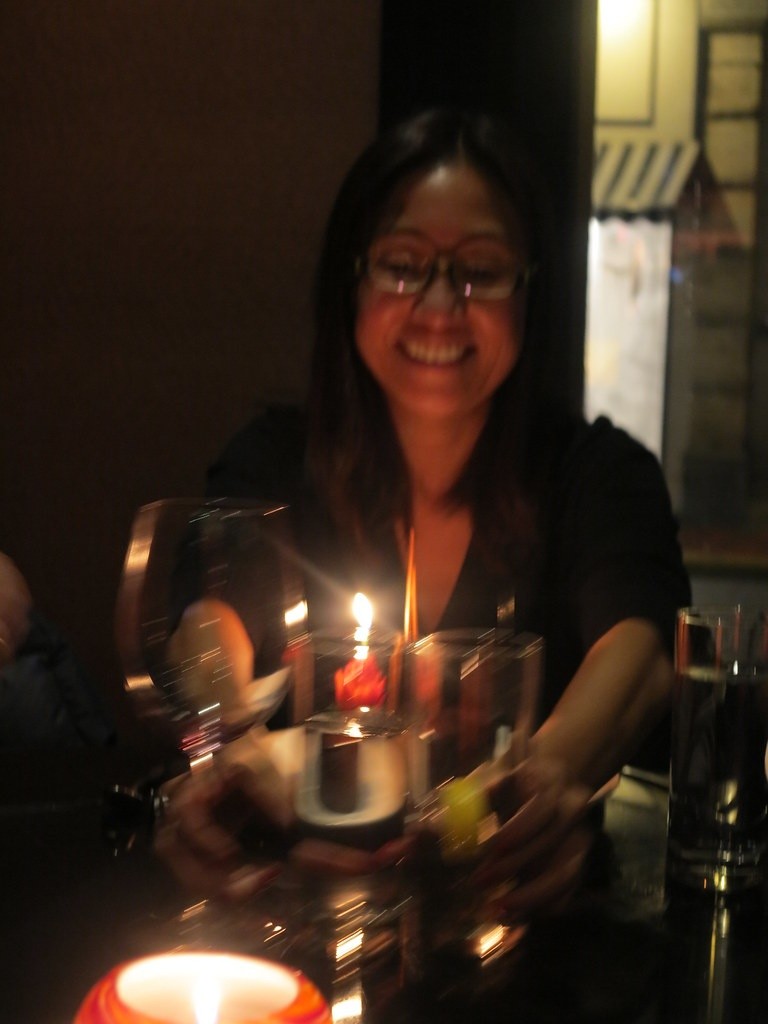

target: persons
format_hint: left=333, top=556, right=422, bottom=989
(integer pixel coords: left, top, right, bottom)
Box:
left=0, top=549, right=113, bottom=745
left=158, top=112, right=690, bottom=926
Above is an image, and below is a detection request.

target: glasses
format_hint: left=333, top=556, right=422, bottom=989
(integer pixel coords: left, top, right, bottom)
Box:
left=346, top=229, right=531, bottom=298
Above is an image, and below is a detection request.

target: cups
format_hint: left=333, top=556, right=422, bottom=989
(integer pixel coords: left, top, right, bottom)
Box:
left=118, top=498, right=300, bottom=777
left=407, top=626, right=543, bottom=805
left=677, top=607, right=768, bottom=880
